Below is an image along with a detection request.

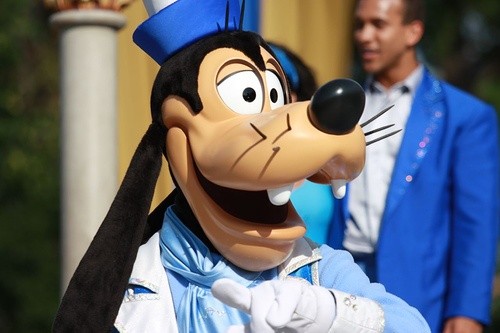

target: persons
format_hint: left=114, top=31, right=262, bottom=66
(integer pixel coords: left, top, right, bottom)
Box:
left=320, top=0, right=500, bottom=333
